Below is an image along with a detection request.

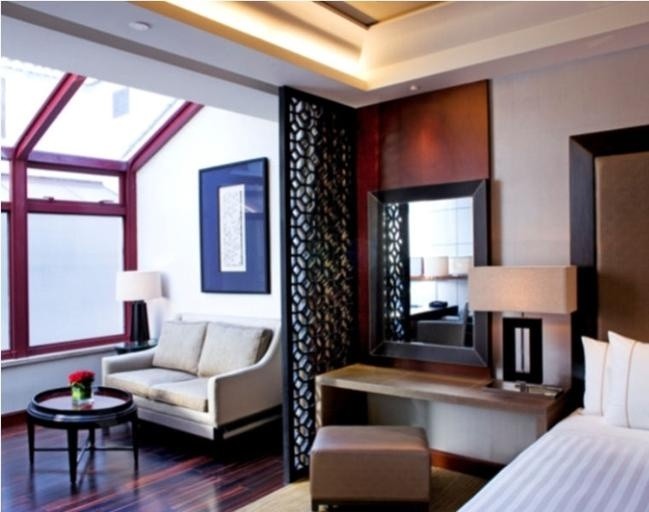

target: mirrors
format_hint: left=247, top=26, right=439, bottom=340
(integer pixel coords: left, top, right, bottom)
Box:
left=365, top=175, right=493, bottom=368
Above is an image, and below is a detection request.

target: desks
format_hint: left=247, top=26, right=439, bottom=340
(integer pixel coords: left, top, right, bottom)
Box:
left=312, top=362, right=570, bottom=442
left=410, top=304, right=459, bottom=341
left=116, top=338, right=158, bottom=353
left=26, top=384, right=141, bottom=495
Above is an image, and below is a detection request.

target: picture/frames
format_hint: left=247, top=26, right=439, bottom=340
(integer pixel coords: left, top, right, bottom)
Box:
left=196, top=155, right=271, bottom=295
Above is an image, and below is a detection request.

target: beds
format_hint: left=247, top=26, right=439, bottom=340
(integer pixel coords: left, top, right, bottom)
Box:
left=454, top=123, right=648, bottom=512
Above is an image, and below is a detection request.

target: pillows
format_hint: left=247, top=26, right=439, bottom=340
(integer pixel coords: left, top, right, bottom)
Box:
left=605, top=330, right=648, bottom=429
left=198, top=320, right=274, bottom=377
left=151, top=318, right=208, bottom=375
left=577, top=335, right=609, bottom=415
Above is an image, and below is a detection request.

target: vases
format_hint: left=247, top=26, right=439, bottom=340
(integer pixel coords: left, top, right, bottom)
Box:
left=70, top=382, right=94, bottom=405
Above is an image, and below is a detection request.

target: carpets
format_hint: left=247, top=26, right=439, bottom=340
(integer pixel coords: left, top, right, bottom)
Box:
left=234, top=464, right=487, bottom=512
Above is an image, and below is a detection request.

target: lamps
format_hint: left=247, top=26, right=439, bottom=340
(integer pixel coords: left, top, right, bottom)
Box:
left=114, top=268, right=162, bottom=343
left=424, top=254, right=450, bottom=307
left=409, top=256, right=423, bottom=308
left=468, top=263, right=578, bottom=385
left=449, top=255, right=473, bottom=309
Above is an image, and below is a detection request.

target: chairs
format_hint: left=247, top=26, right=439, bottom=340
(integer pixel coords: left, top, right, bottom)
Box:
left=416, top=300, right=469, bottom=345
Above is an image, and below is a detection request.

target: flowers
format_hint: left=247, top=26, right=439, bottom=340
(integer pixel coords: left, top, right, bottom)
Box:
left=68, top=370, right=96, bottom=383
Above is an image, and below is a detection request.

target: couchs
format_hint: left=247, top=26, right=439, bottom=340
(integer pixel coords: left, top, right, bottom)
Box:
left=99, top=311, right=282, bottom=452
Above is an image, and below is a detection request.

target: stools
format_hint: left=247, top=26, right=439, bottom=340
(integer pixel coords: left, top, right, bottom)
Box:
left=308, top=422, right=435, bottom=512
